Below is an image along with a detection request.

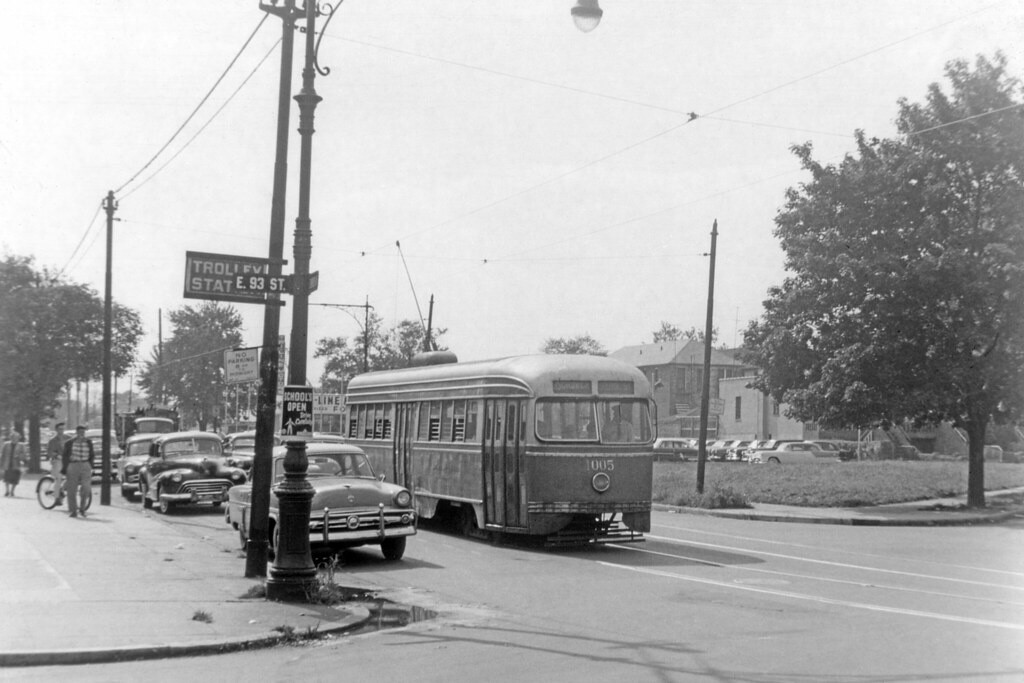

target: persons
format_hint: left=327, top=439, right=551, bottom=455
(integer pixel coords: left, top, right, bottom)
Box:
left=46, top=423, right=73, bottom=506
left=0, top=431, right=28, bottom=497
left=60, top=425, right=96, bottom=518
left=602, top=404, right=636, bottom=442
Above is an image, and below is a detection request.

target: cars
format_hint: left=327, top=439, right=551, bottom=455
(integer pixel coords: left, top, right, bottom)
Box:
left=652, top=437, right=841, bottom=466
left=66, top=430, right=123, bottom=481
left=223, top=443, right=416, bottom=563
left=23, top=430, right=52, bottom=462
left=115, top=434, right=190, bottom=501
left=219, top=432, right=280, bottom=469
left=282, top=433, right=345, bottom=475
left=139, top=431, right=248, bottom=513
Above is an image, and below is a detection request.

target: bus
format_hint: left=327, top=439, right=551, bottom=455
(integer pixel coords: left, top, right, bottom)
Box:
left=340, top=351, right=658, bottom=554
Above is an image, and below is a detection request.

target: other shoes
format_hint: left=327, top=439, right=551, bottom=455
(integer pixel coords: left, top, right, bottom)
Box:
left=80, top=510, right=87, bottom=517
left=69, top=512, right=76, bottom=517
left=56, top=498, right=62, bottom=505
left=5, top=493, right=9, bottom=496
left=60, top=489, right=65, bottom=497
left=11, top=492, right=14, bottom=496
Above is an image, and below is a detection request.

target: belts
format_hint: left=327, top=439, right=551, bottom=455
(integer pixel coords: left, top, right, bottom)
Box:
left=69, top=460, right=89, bottom=463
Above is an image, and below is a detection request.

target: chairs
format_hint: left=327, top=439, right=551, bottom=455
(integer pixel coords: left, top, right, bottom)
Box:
left=316, top=463, right=339, bottom=476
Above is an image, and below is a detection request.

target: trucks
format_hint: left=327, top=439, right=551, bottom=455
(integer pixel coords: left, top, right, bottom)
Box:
left=114, top=408, right=180, bottom=452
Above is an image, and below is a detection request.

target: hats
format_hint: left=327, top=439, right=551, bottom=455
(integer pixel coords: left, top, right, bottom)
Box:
left=77, top=425, right=86, bottom=430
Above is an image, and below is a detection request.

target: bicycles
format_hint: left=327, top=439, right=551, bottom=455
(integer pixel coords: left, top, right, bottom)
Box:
left=35, top=473, right=92, bottom=511
